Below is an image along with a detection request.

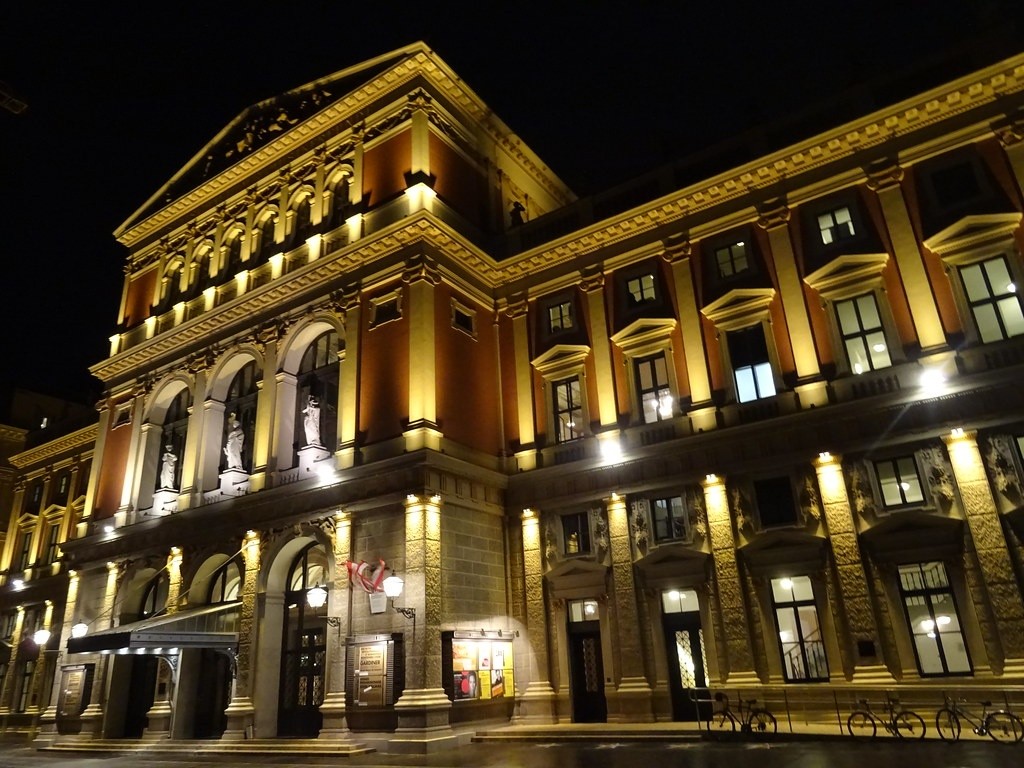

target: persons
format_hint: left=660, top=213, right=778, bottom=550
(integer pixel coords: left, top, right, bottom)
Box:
left=302, top=395, right=321, bottom=446
left=224, top=413, right=244, bottom=470
left=161, top=445, right=177, bottom=490
left=495, top=670, right=502, bottom=685
left=469, top=671, right=477, bottom=698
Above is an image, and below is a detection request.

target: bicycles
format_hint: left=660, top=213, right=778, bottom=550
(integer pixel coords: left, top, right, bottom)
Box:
left=707, top=693, right=777, bottom=735
left=935, top=692, right=1024, bottom=746
left=846, top=691, right=927, bottom=740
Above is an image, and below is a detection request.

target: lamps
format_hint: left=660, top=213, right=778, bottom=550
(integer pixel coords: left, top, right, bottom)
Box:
left=481, top=629, right=485, bottom=636
left=383, top=569, right=416, bottom=628
left=71, top=619, right=89, bottom=638
left=307, top=581, right=341, bottom=637
left=516, top=631, right=519, bottom=637
left=33, top=626, right=63, bottom=660
left=497, top=630, right=503, bottom=637
